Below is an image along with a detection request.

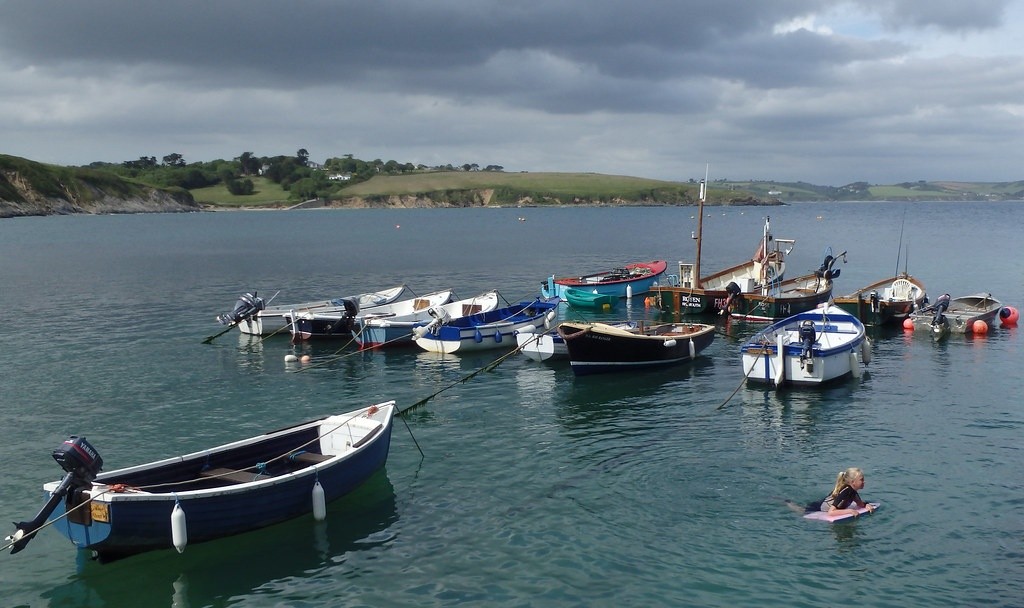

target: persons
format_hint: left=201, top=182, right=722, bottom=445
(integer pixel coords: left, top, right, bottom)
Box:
left=813, top=467, right=875, bottom=517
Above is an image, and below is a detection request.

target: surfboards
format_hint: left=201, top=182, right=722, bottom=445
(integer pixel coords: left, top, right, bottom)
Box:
left=801, top=501, right=883, bottom=524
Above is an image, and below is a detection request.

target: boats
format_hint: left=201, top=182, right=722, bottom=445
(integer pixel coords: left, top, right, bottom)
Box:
left=644, top=163, right=795, bottom=316
left=541, top=261, right=667, bottom=302
left=834, top=273, right=925, bottom=326
left=740, top=304, right=865, bottom=385
left=412, top=294, right=560, bottom=353
left=909, top=292, right=1005, bottom=333
left=557, top=322, right=716, bottom=366
left=720, top=247, right=847, bottom=324
left=200, top=286, right=405, bottom=344
left=326, top=289, right=499, bottom=345
left=282, top=288, right=453, bottom=342
left=565, top=288, right=619, bottom=311
left=512, top=320, right=637, bottom=362
left=4, top=401, right=396, bottom=564
